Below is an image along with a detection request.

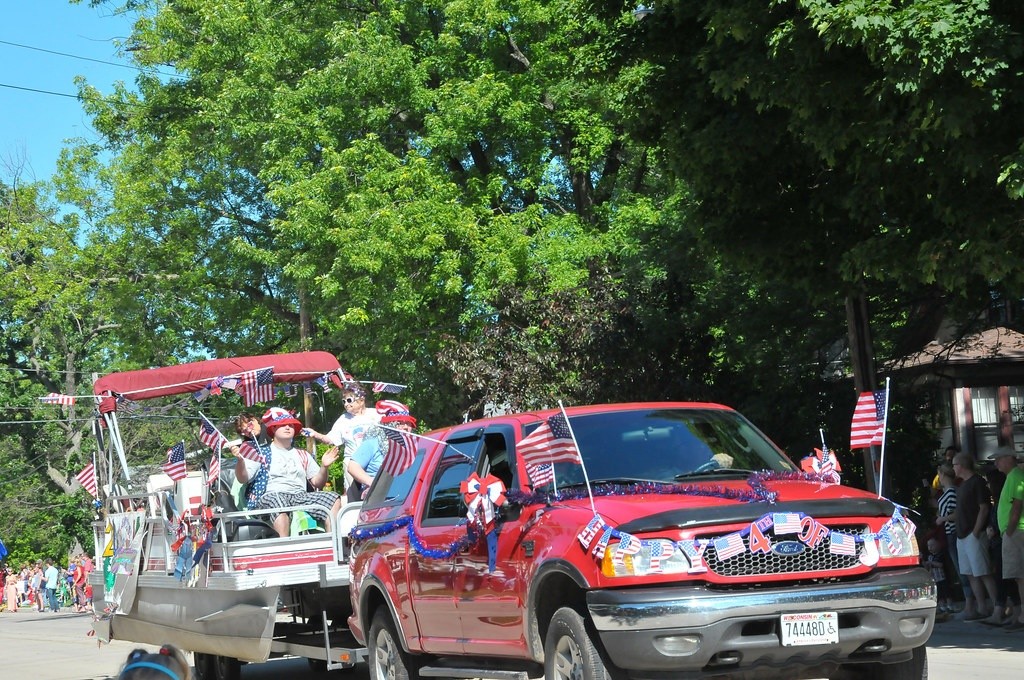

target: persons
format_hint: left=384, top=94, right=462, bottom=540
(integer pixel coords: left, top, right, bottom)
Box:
left=988, top=445, right=1024, bottom=633
left=1, top=541, right=95, bottom=616
left=974, top=462, right=1023, bottom=629
left=933, top=445, right=957, bottom=494
left=935, top=464, right=974, bottom=620
left=234, top=380, right=420, bottom=540
left=952, top=451, right=1009, bottom=623
left=117, top=643, right=193, bottom=680
left=922, top=538, right=951, bottom=614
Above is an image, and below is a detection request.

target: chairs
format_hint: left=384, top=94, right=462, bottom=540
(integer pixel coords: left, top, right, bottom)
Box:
left=238, top=479, right=325, bottom=539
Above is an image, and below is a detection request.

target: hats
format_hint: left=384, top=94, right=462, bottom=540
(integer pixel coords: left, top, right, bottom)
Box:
left=262, top=407, right=302, bottom=439
left=988, top=446, right=1017, bottom=460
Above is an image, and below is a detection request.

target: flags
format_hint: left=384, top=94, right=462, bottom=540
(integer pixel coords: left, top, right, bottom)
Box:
left=828, top=531, right=856, bottom=557
left=849, top=388, right=888, bottom=448
left=39, top=390, right=74, bottom=405
left=525, top=460, right=555, bottom=490
left=75, top=461, right=98, bottom=500
left=713, top=532, right=747, bottom=561
left=772, top=511, right=802, bottom=535
left=162, top=366, right=276, bottom=487
left=821, top=445, right=833, bottom=479
left=515, top=411, right=584, bottom=466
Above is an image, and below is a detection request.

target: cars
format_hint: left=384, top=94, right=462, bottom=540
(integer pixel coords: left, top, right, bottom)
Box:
left=346, top=401, right=939, bottom=680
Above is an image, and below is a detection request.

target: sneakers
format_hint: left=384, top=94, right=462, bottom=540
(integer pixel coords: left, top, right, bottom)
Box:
left=1004, top=620, right=1024, bottom=634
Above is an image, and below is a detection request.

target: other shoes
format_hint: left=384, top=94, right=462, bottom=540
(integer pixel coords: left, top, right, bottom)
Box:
left=954, top=611, right=977, bottom=620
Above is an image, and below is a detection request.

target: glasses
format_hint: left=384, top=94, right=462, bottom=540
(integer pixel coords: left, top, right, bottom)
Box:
left=952, top=463, right=961, bottom=466
left=376, top=399, right=416, bottom=428
left=342, top=397, right=361, bottom=405
left=242, top=419, right=254, bottom=436
